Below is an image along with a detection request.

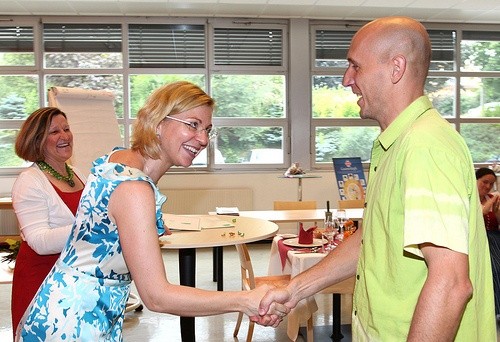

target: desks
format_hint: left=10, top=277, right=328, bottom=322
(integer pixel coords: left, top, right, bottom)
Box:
left=208, top=207, right=364, bottom=291
left=277, top=174, right=322, bottom=201
left=157, top=214, right=280, bottom=342
left=268, top=233, right=356, bottom=342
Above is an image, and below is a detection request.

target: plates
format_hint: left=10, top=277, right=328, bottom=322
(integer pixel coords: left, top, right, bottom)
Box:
left=282, top=238, right=328, bottom=247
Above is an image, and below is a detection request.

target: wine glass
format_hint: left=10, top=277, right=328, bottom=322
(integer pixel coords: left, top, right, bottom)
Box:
left=324, top=220, right=335, bottom=250
left=337, top=210, right=347, bottom=241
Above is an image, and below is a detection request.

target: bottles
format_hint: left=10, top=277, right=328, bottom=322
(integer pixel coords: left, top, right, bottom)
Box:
left=326, top=201, right=332, bottom=222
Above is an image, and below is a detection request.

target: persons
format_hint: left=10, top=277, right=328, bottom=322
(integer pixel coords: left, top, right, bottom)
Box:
left=17, top=80, right=290, bottom=342
left=11, top=107, right=88, bottom=342
left=249, top=17, right=500, bottom=342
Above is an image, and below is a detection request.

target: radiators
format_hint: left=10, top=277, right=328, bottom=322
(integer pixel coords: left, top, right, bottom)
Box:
left=159, top=188, right=253, bottom=215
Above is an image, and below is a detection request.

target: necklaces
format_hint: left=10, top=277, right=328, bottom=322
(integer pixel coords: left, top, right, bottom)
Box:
left=39, top=162, right=76, bottom=188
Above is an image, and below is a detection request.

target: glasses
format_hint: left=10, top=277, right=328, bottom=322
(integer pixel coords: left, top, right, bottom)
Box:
left=165, top=116, right=218, bottom=142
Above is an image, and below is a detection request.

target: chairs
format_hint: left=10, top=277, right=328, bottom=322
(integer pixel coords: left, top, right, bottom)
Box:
left=233, top=243, right=318, bottom=342
left=273, top=200, right=317, bottom=210
left=338, top=199, right=365, bottom=209
